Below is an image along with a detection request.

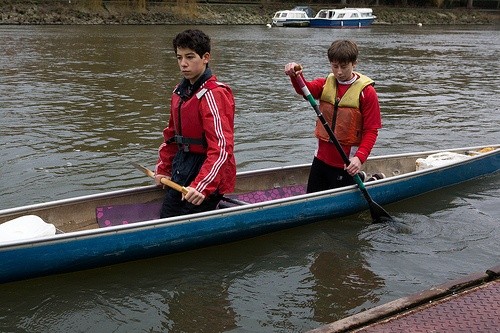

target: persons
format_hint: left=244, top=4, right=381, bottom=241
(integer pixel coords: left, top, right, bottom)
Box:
left=284, top=39, right=382, bottom=194
left=153, top=28, right=238, bottom=220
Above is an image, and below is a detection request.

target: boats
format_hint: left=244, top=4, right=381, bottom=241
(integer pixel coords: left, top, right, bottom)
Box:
left=311, top=8, right=378, bottom=27
left=0, top=144, right=500, bottom=275
left=271, top=10, right=313, bottom=27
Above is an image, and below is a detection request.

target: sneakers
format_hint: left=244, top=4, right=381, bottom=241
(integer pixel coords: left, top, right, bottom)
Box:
left=356, top=171, right=365, bottom=183
left=368, top=171, right=384, bottom=182
left=219, top=197, right=251, bottom=209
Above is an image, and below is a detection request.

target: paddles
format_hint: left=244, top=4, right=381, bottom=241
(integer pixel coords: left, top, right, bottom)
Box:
left=286, top=64, right=392, bottom=219
left=129, top=160, right=187, bottom=195
